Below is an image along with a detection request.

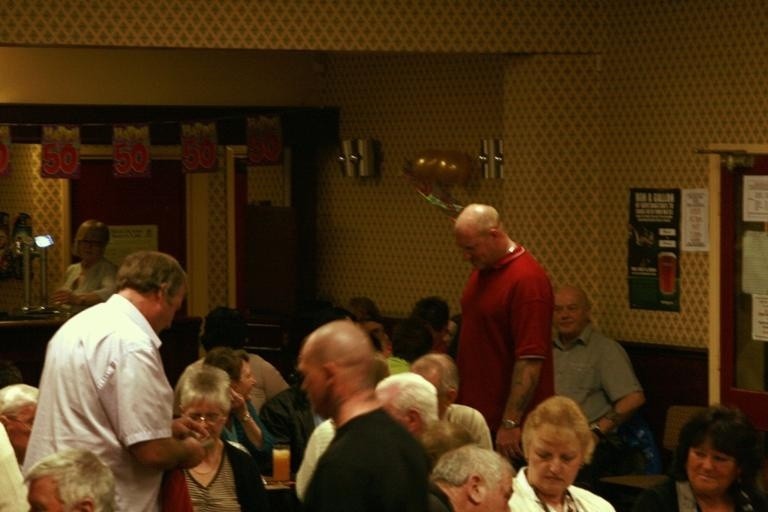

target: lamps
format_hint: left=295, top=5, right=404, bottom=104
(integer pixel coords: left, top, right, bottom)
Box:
left=334, top=136, right=380, bottom=180
left=476, top=135, right=507, bottom=180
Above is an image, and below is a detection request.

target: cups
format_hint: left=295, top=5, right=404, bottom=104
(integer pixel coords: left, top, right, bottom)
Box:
left=657, top=252, right=677, bottom=295
left=271, top=443, right=292, bottom=482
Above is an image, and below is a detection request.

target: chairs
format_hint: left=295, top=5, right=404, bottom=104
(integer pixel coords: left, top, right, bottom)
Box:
left=595, top=404, right=708, bottom=511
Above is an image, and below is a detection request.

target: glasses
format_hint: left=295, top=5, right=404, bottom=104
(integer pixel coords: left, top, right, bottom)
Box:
left=188, top=412, right=225, bottom=425
left=7, top=415, right=33, bottom=430
